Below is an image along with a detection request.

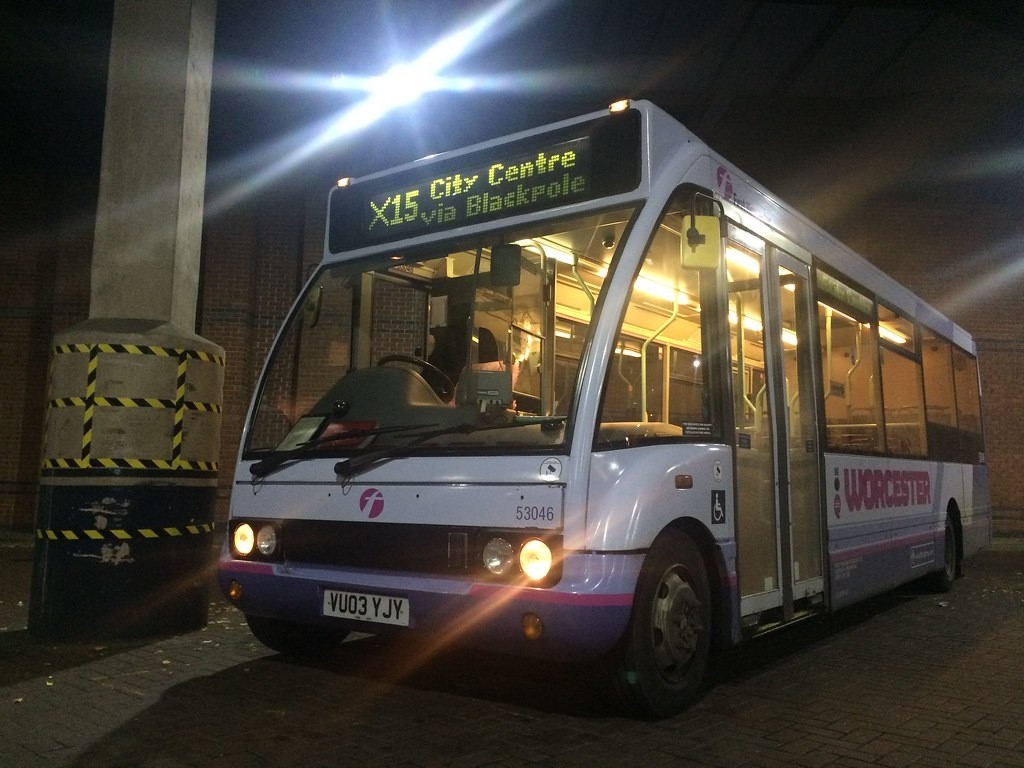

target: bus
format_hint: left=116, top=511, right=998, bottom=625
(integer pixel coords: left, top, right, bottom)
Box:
left=213, top=98, right=995, bottom=723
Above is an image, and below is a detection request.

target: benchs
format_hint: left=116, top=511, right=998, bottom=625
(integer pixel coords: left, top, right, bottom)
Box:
left=827, top=404, right=962, bottom=453
